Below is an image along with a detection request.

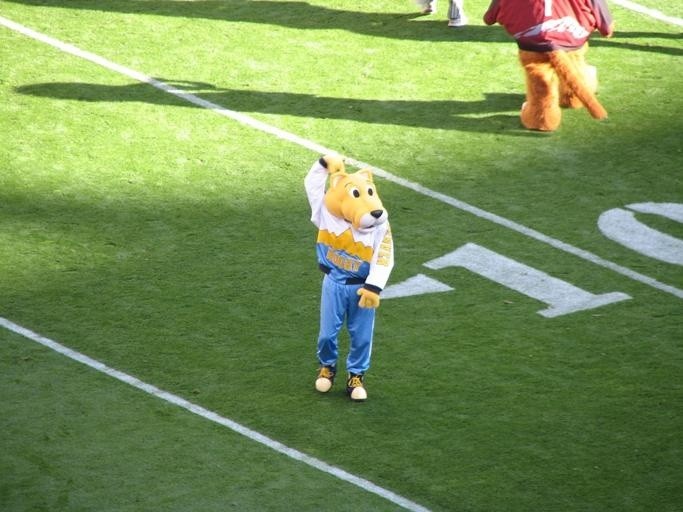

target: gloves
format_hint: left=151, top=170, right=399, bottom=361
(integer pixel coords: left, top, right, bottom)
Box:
left=320, top=151, right=346, bottom=176
left=357, top=282, right=379, bottom=310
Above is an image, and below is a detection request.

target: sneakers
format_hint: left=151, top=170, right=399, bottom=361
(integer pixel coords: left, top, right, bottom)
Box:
left=313, top=362, right=336, bottom=393
left=345, top=372, right=368, bottom=400
left=420, top=6, right=469, bottom=28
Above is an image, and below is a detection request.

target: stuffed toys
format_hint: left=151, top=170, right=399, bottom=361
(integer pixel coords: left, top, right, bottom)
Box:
left=482, top=1, right=613, bottom=133
left=302, top=151, right=395, bottom=405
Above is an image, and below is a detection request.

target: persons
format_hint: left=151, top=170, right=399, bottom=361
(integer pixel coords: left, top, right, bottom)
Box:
left=416, top=0, right=469, bottom=28
